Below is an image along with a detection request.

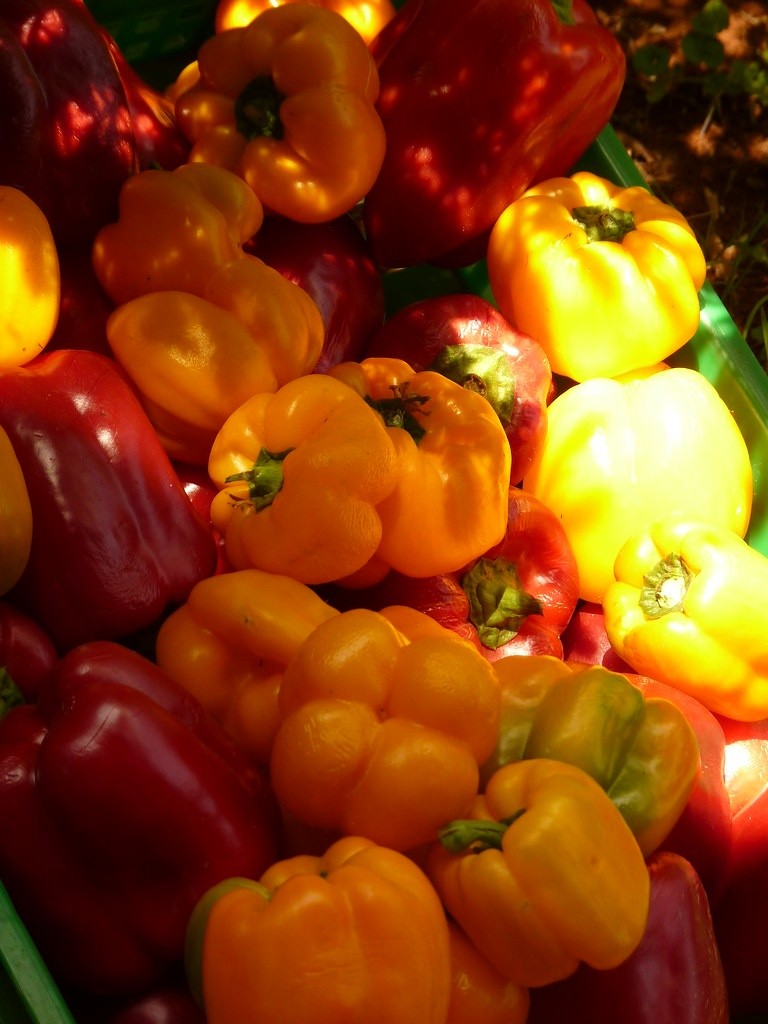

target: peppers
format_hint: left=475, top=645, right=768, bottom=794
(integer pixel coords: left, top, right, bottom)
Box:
left=0, top=0, right=768, bottom=1024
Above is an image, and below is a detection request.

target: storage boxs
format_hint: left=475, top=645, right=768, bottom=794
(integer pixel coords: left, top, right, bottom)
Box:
left=0, top=1, right=767, bottom=1024
